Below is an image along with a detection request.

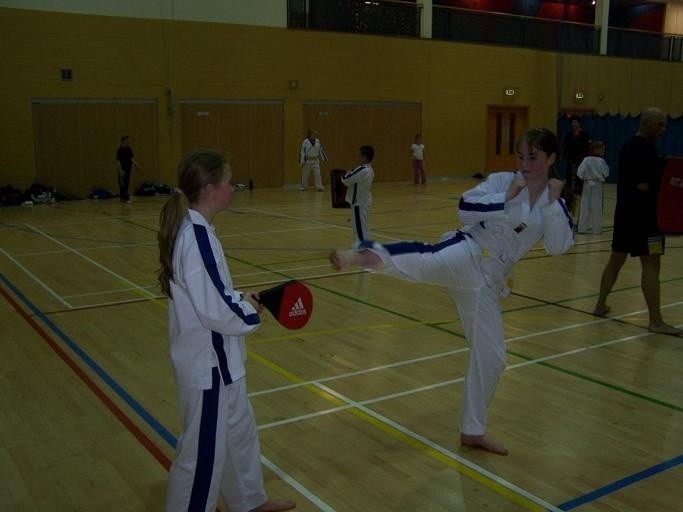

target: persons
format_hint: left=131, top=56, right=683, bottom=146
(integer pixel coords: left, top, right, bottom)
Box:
left=577, top=139, right=610, bottom=236
left=300, top=128, right=329, bottom=190
left=331, top=128, right=576, bottom=456
left=163, top=151, right=294, bottom=511
left=410, top=135, right=428, bottom=188
left=593, top=108, right=683, bottom=335
left=116, top=136, right=140, bottom=204
left=565, top=117, right=590, bottom=193
